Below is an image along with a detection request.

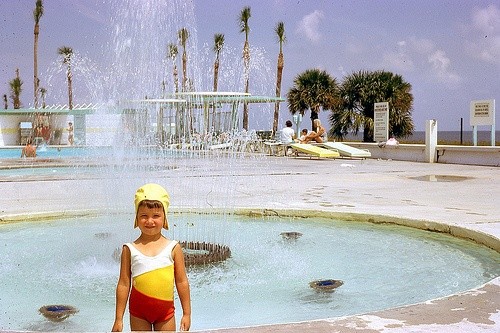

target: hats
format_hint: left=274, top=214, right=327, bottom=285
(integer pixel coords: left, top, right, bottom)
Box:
left=134, top=184, right=170, bottom=230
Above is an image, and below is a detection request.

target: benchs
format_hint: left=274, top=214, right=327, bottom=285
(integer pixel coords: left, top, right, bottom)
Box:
left=168, top=139, right=371, bottom=161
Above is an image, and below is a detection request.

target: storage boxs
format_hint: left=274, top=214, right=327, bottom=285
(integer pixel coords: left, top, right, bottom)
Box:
left=18, top=121, right=32, bottom=145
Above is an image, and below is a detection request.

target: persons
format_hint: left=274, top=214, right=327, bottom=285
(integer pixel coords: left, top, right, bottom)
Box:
left=20, top=107, right=329, bottom=158
left=112, top=183, right=191, bottom=332
